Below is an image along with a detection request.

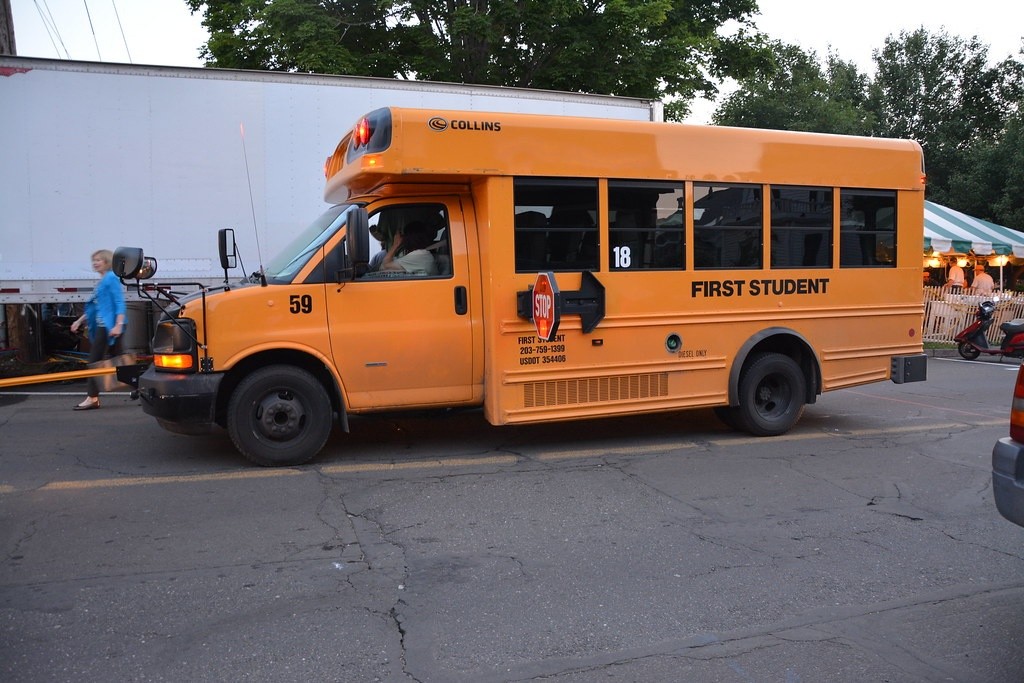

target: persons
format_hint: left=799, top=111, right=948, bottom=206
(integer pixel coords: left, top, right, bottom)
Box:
left=366, top=222, right=438, bottom=275
left=945, top=258, right=964, bottom=293
left=972, top=264, right=995, bottom=296
left=71, top=250, right=139, bottom=410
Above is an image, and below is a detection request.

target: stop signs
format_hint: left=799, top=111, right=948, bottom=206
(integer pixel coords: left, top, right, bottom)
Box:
left=532, top=269, right=560, bottom=342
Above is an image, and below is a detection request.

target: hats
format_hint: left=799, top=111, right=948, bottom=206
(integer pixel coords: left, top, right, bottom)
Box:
left=973, top=265, right=984, bottom=270
left=950, top=257, right=957, bottom=262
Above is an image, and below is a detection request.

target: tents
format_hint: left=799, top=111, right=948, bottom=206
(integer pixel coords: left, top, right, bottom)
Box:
left=861, top=198, right=1024, bottom=294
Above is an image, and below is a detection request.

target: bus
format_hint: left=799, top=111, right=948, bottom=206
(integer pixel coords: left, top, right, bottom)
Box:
left=112, top=107, right=928, bottom=471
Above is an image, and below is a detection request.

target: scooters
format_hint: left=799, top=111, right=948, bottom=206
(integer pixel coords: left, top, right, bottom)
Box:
left=954, top=300, right=1024, bottom=364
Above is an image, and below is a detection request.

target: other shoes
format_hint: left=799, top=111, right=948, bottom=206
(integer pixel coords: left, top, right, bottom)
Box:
left=73, top=400, right=99, bottom=409
